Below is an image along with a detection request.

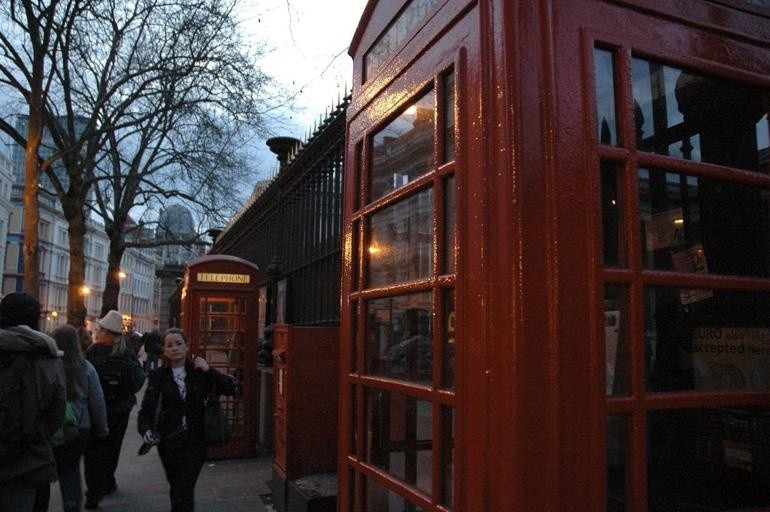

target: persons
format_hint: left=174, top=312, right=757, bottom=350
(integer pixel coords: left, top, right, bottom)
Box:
left=78, top=324, right=165, bottom=378
left=0, top=289, right=66, bottom=511
left=136, top=325, right=236, bottom=511
left=379, top=305, right=432, bottom=359
left=48, top=324, right=110, bottom=512
left=84, top=309, right=145, bottom=495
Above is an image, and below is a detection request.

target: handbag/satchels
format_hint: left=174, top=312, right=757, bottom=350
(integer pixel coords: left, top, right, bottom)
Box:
left=203, top=400, right=231, bottom=449
left=52, top=402, right=81, bottom=453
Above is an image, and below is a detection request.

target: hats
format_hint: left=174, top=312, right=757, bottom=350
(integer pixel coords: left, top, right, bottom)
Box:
left=96, top=310, right=123, bottom=335
left=0, top=292, right=41, bottom=320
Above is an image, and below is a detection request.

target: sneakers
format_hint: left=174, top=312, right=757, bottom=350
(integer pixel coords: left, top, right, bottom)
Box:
left=84, top=480, right=118, bottom=511
